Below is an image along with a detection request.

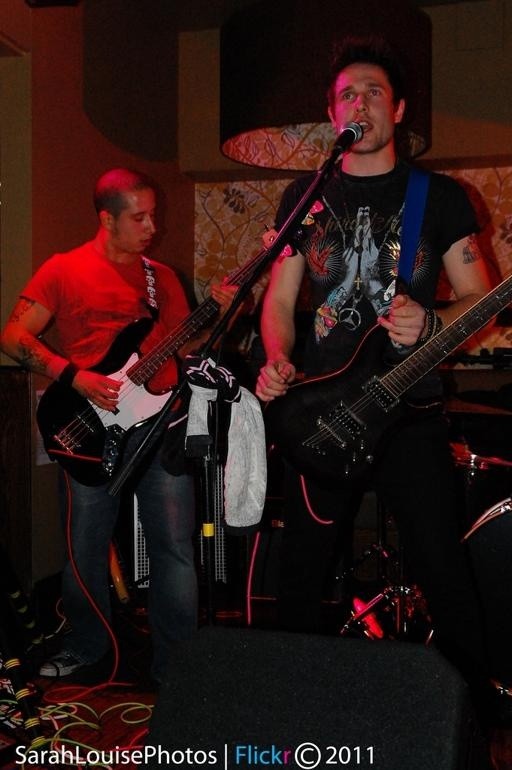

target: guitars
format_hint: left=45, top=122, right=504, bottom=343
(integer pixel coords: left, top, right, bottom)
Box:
left=262, top=276, right=512, bottom=488
left=36, top=201, right=326, bottom=489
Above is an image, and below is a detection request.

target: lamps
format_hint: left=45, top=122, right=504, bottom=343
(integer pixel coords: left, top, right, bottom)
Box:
left=220, top=2, right=432, bottom=172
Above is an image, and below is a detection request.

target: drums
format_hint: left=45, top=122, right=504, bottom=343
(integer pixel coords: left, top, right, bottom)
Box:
left=447, top=495, right=511, bottom=699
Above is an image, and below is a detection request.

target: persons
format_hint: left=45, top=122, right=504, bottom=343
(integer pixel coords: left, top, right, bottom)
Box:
left=0, top=168, right=245, bottom=687
left=255, top=37, right=496, bottom=633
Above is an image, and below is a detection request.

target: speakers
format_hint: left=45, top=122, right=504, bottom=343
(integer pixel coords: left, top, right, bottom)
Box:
left=136, top=625, right=493, bottom=770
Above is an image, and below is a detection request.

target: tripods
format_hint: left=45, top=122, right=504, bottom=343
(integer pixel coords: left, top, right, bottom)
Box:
left=326, top=486, right=438, bottom=644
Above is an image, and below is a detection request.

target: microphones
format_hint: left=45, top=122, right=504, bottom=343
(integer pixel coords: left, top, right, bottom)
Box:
left=331, top=121, right=363, bottom=159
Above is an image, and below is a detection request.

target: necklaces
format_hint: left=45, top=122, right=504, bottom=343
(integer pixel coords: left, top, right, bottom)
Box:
left=339, top=173, right=384, bottom=292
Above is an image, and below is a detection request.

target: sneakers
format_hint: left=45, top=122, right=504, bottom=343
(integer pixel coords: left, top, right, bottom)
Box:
left=40, top=649, right=95, bottom=676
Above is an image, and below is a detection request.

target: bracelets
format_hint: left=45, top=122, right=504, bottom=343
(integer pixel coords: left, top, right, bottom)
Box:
left=59, top=363, right=80, bottom=386
left=422, top=306, right=442, bottom=342
